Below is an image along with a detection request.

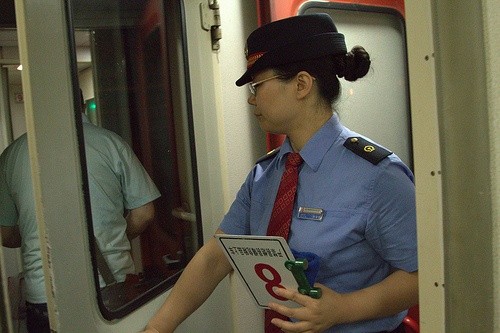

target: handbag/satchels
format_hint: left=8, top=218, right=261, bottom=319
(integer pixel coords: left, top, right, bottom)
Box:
left=101, top=280, right=132, bottom=312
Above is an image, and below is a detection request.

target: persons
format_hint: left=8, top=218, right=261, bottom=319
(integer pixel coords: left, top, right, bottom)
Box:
left=0, top=87, right=161, bottom=333
left=137, top=13, right=420, bottom=333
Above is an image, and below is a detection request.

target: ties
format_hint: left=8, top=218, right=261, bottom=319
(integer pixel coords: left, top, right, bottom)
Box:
left=265, top=153, right=299, bottom=333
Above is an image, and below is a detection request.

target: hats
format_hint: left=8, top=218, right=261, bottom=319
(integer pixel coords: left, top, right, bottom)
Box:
left=236, top=13, right=346, bottom=86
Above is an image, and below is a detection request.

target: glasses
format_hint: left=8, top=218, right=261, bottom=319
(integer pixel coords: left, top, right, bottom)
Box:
left=249, top=75, right=315, bottom=95
